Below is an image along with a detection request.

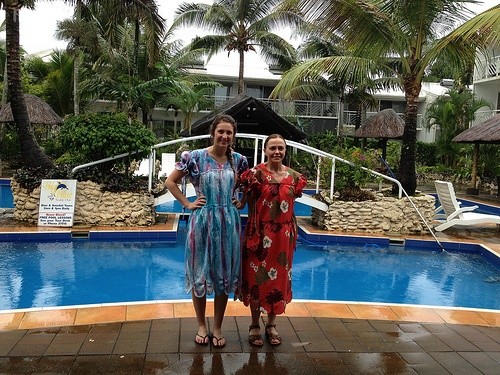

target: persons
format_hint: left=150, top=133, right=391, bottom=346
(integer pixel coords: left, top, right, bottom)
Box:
left=240, top=135, right=309, bottom=348
left=164, top=114, right=248, bottom=348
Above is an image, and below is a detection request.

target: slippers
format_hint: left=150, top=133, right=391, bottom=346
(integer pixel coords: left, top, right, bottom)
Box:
left=195, top=333, right=209, bottom=346
left=211, top=334, right=226, bottom=349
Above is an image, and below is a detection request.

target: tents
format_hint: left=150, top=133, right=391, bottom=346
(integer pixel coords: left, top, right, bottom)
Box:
left=178, top=94, right=307, bottom=141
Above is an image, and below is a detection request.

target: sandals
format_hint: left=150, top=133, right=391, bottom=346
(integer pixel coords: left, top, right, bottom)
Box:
left=265, top=324, right=282, bottom=345
left=249, top=325, right=263, bottom=346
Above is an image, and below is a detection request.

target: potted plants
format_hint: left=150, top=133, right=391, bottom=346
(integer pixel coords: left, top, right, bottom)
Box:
left=324, top=105, right=336, bottom=116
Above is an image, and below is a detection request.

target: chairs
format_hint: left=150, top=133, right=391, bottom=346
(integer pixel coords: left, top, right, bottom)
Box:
left=433, top=179, right=500, bottom=232
